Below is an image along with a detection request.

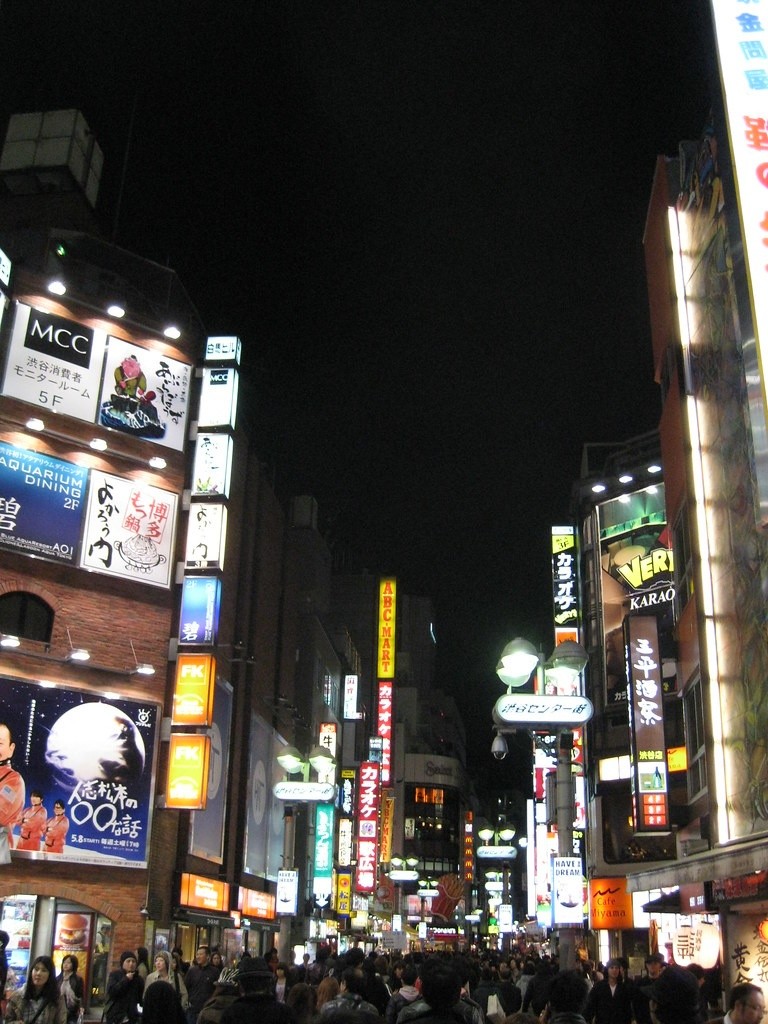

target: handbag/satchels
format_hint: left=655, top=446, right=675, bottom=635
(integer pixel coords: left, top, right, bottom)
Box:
left=486, top=987, right=506, bottom=1024
left=101, top=991, right=114, bottom=1014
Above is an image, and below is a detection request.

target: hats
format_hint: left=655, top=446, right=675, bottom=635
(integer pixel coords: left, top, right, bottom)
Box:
left=638, top=966, right=704, bottom=1012
left=154, top=950, right=171, bottom=969
left=120, top=951, right=137, bottom=970
left=232, top=957, right=273, bottom=982
left=212, top=967, right=239, bottom=989
left=241, top=951, right=251, bottom=960
left=645, top=953, right=667, bottom=966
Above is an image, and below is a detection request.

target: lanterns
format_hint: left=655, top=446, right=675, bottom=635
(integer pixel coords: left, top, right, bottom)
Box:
left=672, top=925, right=694, bottom=967
left=690, top=921, right=719, bottom=969
left=759, top=916, right=768, bottom=946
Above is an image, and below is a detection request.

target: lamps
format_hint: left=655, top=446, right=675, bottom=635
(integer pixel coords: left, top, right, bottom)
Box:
left=129, top=663, right=155, bottom=675
left=27, top=417, right=45, bottom=431
left=64, top=648, right=91, bottom=662
left=0, top=634, right=21, bottom=650
left=149, top=457, right=167, bottom=469
left=90, top=438, right=107, bottom=451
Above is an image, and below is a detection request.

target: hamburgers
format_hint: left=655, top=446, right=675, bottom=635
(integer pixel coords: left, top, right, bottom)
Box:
left=59, top=914, right=88, bottom=947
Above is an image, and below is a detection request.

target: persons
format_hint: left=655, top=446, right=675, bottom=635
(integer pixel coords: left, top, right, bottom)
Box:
left=0, top=718, right=69, bottom=864
left=0, top=930, right=85, bottom=1024
left=704, top=983, right=765, bottom=1024
left=102, top=945, right=724, bottom=1024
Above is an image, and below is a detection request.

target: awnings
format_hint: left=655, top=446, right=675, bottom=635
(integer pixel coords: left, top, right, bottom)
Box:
left=171, top=907, right=235, bottom=929
left=241, top=917, right=280, bottom=933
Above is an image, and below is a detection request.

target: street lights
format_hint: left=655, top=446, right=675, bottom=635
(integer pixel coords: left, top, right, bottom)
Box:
left=493, top=636, right=595, bottom=972
left=275, top=741, right=338, bottom=965
left=484, top=867, right=512, bottom=948
left=387, top=854, right=419, bottom=957
left=474, top=816, right=517, bottom=955
left=416, top=876, right=441, bottom=953
